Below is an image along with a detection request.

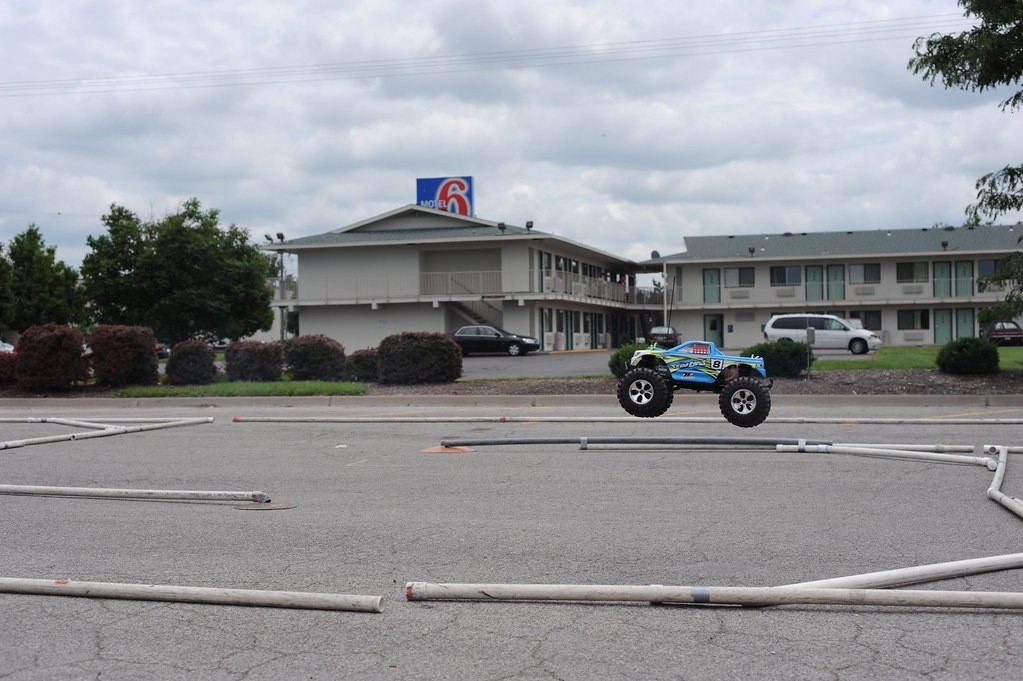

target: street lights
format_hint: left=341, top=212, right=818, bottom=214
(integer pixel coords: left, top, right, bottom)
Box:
left=265, top=232, right=286, bottom=366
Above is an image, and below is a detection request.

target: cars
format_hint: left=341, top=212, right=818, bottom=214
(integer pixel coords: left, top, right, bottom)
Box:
left=81, top=343, right=171, bottom=378
left=649, top=326, right=682, bottom=348
left=449, top=324, right=540, bottom=358
left=988, top=321, right=1023, bottom=346
left=0, top=341, right=17, bottom=364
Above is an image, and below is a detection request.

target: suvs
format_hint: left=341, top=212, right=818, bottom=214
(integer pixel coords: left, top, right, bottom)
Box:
left=763, top=313, right=882, bottom=355
left=617, top=341, right=774, bottom=429
